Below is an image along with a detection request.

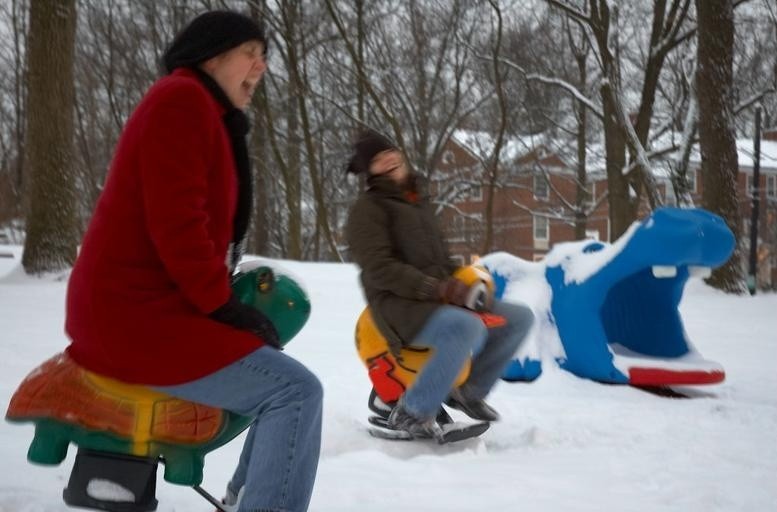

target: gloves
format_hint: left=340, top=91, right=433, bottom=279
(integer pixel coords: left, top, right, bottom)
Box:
left=434, top=279, right=468, bottom=306
left=207, top=295, right=281, bottom=351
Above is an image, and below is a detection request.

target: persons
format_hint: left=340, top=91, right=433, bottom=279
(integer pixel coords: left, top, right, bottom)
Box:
left=341, top=129, right=535, bottom=446
left=62, top=9, right=327, bottom=511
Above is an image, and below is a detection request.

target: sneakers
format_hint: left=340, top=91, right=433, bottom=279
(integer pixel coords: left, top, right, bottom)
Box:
left=442, top=384, right=501, bottom=421
left=385, top=404, right=445, bottom=445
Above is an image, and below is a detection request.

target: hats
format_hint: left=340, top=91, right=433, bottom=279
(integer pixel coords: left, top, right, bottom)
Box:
left=345, top=132, right=398, bottom=173
left=160, top=10, right=268, bottom=70
